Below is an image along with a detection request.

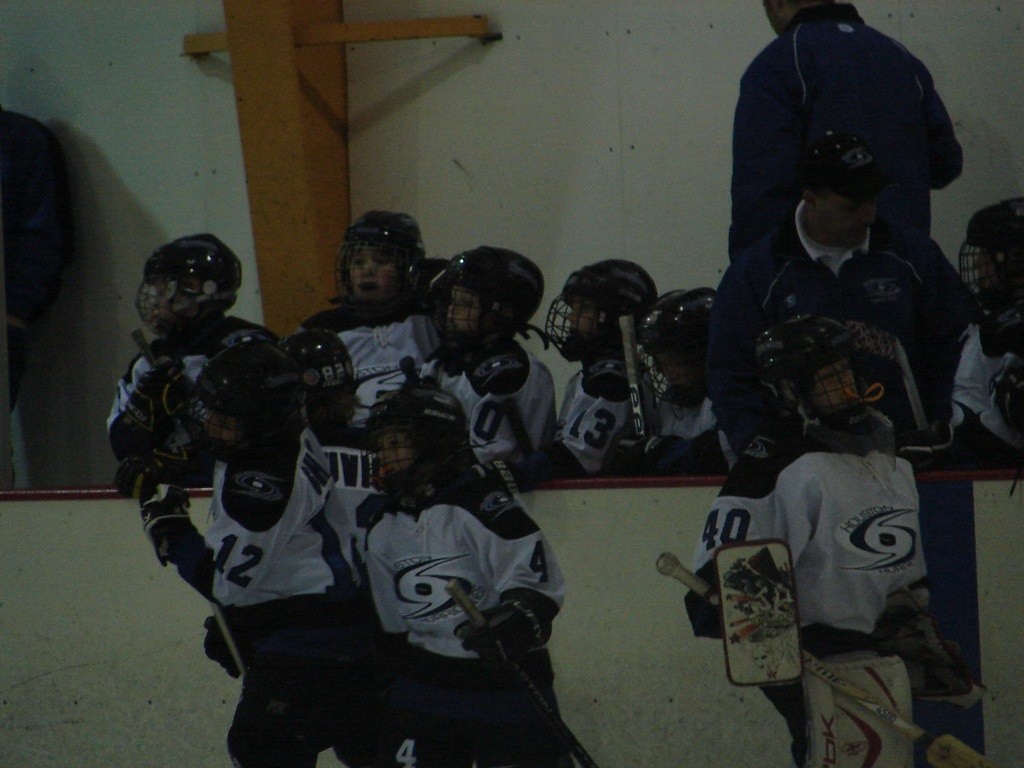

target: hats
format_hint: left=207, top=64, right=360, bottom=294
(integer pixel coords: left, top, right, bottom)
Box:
left=796, top=130, right=902, bottom=211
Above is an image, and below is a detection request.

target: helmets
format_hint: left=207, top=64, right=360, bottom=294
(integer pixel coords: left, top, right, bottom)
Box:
left=544, top=258, right=658, bottom=364
left=749, top=317, right=883, bottom=425
left=181, top=341, right=306, bottom=465
left=361, top=386, right=468, bottom=514
left=429, top=246, right=550, bottom=351
left=334, top=210, right=425, bottom=321
left=276, top=329, right=357, bottom=426
left=133, top=232, right=241, bottom=337
left=637, top=286, right=718, bottom=407
left=959, top=198, right=1024, bottom=311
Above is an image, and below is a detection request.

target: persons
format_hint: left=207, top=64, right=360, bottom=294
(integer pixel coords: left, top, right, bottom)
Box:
left=694, top=129, right=998, bottom=768
left=103, top=196, right=1024, bottom=494
left=684, top=310, right=972, bottom=768
left=362, top=383, right=574, bottom=768
left=137, top=327, right=384, bottom=768
left=0, top=109, right=77, bottom=489
left=727, top=0, right=962, bottom=262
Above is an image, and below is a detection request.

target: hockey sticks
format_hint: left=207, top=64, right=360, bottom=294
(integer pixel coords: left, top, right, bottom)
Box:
left=130, top=326, right=210, bottom=487
left=443, top=577, right=600, bottom=768
left=207, top=602, right=249, bottom=681
left=620, top=312, right=647, bottom=438
left=840, top=318, right=930, bottom=431
left=653, top=551, right=997, bottom=768
left=500, top=395, right=533, bottom=461
left=398, top=356, right=421, bottom=386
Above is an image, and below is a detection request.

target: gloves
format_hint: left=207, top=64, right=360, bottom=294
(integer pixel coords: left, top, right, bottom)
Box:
left=455, top=587, right=559, bottom=671
left=609, top=427, right=723, bottom=478
left=109, top=363, right=190, bottom=463
left=896, top=419, right=953, bottom=472
left=979, top=303, right=1024, bottom=358
left=485, top=459, right=522, bottom=497
left=988, top=364, right=1024, bottom=440
left=372, top=630, right=411, bottom=690
left=166, top=528, right=215, bottom=596
left=202, top=614, right=242, bottom=682
